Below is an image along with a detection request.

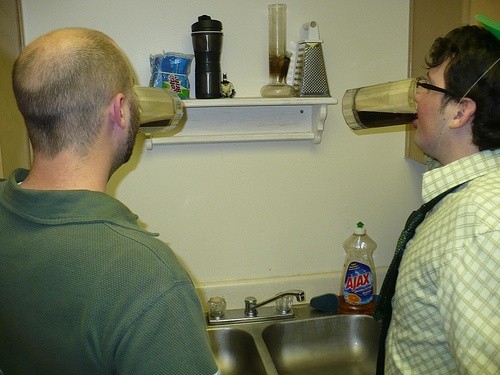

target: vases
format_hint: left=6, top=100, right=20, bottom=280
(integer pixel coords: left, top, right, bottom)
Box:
left=260, top=4, right=294, bottom=97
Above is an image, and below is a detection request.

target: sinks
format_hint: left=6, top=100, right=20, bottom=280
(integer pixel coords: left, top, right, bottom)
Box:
left=258, top=313, right=388, bottom=375
left=204, top=325, right=269, bottom=375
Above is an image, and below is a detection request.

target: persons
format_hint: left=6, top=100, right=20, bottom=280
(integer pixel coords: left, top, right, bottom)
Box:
left=375, top=14, right=500, bottom=375
left=0, top=28, right=220, bottom=375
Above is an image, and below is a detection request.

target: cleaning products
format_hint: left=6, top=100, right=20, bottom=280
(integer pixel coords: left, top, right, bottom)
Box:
left=340, top=222, right=376, bottom=316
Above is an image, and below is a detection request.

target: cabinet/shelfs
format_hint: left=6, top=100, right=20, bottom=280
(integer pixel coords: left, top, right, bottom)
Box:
left=405, top=0, right=500, bottom=165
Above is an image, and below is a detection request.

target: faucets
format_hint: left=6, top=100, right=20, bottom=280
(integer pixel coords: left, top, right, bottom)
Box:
left=245, top=289, right=305, bottom=321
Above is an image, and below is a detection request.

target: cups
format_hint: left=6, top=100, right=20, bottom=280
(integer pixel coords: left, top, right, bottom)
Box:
left=133, top=85, right=183, bottom=133
left=343, top=76, right=429, bottom=135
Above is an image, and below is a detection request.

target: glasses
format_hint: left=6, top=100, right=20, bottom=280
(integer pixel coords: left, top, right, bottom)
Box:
left=412, top=75, right=462, bottom=104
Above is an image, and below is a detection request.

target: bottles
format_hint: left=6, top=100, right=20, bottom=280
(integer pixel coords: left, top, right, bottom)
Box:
left=336, top=222, right=378, bottom=316
left=191, top=15, right=224, bottom=99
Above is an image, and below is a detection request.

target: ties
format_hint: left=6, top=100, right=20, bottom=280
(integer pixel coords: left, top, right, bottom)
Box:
left=375, top=184, right=460, bottom=375
